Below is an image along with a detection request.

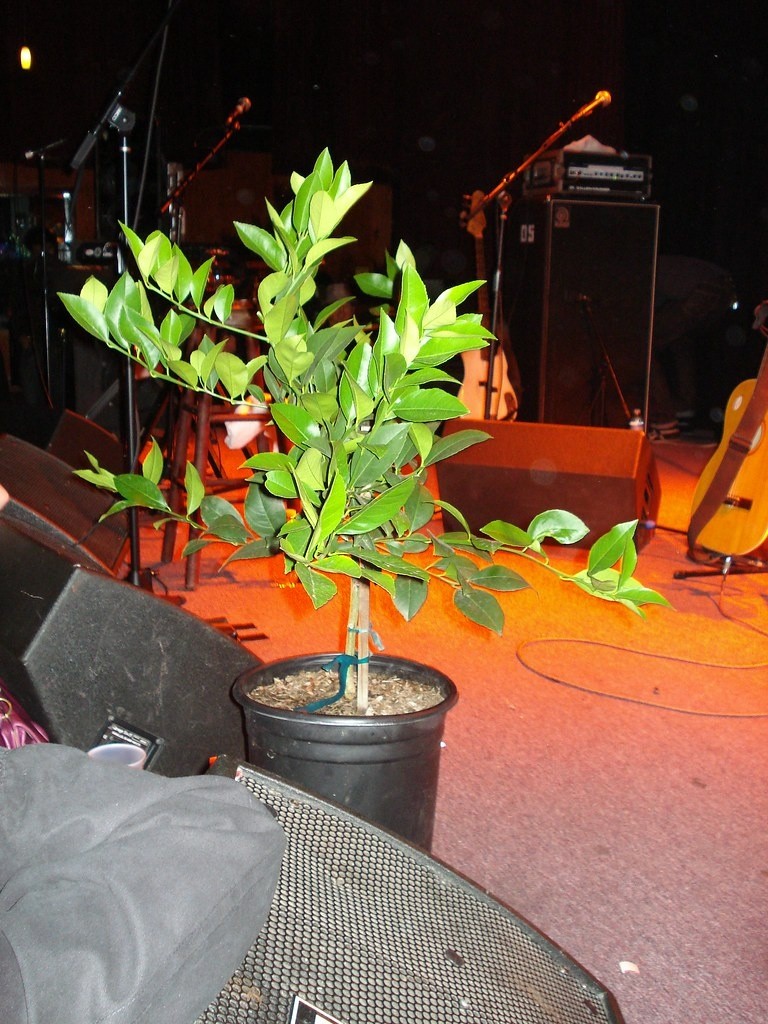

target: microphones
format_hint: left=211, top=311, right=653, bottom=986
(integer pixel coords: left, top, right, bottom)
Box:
left=564, top=289, right=592, bottom=304
left=574, top=91, right=612, bottom=122
left=227, top=98, right=252, bottom=125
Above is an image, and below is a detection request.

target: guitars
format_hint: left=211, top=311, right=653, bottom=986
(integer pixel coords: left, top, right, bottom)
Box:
left=686, top=298, right=768, bottom=573
left=436, top=190, right=524, bottom=423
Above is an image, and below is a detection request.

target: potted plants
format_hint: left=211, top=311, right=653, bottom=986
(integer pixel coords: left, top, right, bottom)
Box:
left=55, top=146, right=675, bottom=852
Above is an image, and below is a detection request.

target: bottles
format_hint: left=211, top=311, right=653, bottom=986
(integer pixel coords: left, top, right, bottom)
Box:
left=629, top=410, right=644, bottom=430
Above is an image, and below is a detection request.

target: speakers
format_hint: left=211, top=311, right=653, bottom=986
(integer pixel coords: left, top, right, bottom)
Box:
left=436, top=419, right=661, bottom=555
left=507, top=197, right=662, bottom=439
left=0, top=410, right=628, bottom=1024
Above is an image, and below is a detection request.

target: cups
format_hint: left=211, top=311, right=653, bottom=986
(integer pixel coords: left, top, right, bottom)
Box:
left=88, top=744, right=146, bottom=770
